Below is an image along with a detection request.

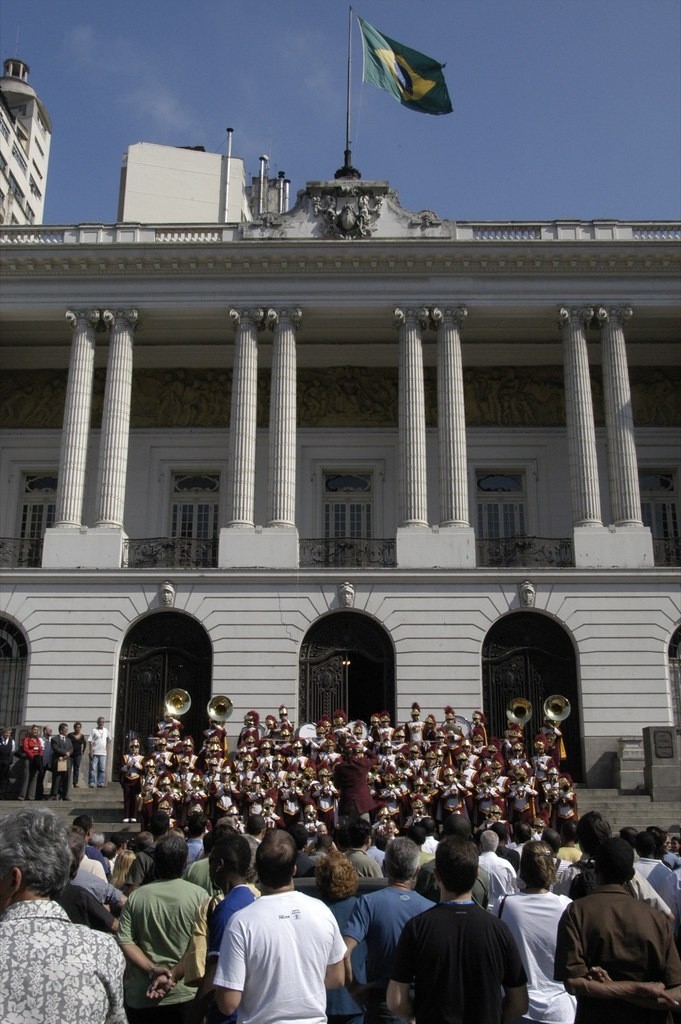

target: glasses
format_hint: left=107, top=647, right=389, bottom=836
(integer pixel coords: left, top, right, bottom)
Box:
left=665, top=840, right=671, bottom=846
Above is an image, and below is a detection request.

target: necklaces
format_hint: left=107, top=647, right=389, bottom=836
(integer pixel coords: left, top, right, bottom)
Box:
left=439, top=900, right=475, bottom=906
left=388, top=881, right=409, bottom=888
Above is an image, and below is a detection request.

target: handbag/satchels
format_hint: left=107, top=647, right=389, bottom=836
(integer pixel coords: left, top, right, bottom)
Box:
left=57, top=756, right=68, bottom=771
left=14, top=745, right=29, bottom=759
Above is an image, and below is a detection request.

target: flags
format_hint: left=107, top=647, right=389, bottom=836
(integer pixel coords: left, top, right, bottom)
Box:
left=357, top=14, right=454, bottom=117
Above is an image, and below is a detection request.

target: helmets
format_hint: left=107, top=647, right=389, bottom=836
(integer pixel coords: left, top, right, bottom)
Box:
left=353, top=702, right=573, bottom=790
left=377, top=803, right=390, bottom=817
left=489, top=796, right=505, bottom=814
left=411, top=794, right=427, bottom=808
left=533, top=810, right=549, bottom=831
left=128, top=705, right=347, bottom=816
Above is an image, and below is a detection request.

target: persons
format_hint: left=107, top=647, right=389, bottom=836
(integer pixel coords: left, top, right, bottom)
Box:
left=340, top=586, right=353, bottom=606
left=0, top=699, right=681, bottom=1024
left=520, top=586, right=535, bottom=604
left=160, top=585, right=174, bottom=603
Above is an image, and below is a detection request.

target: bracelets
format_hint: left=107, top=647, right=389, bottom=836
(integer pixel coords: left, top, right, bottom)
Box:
left=148, top=966, right=155, bottom=980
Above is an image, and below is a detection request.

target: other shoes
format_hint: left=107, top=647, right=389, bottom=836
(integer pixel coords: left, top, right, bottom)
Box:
left=89, top=784, right=95, bottom=788
left=73, top=784, right=80, bottom=788
left=122, top=818, right=129, bottom=822
left=61, top=797, right=72, bottom=801
left=98, top=784, right=107, bottom=787
left=48, top=795, right=57, bottom=801
left=131, top=818, right=137, bottom=822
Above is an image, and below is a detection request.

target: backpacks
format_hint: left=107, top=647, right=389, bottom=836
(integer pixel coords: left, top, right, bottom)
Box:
left=568, top=861, right=638, bottom=902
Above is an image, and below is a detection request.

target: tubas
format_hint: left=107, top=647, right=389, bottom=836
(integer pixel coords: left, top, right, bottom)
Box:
left=206, top=695, right=233, bottom=722
left=163, top=687, right=192, bottom=719
left=543, top=695, right=572, bottom=722
left=506, top=697, right=533, bottom=725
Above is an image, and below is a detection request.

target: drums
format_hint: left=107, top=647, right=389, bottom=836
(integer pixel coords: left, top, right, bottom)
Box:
left=295, top=722, right=318, bottom=739
left=346, top=720, right=368, bottom=742
left=441, top=715, right=472, bottom=739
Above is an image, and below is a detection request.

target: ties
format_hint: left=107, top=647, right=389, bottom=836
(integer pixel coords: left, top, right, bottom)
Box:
left=4, top=739, right=8, bottom=745
left=62, top=737, right=64, bottom=742
